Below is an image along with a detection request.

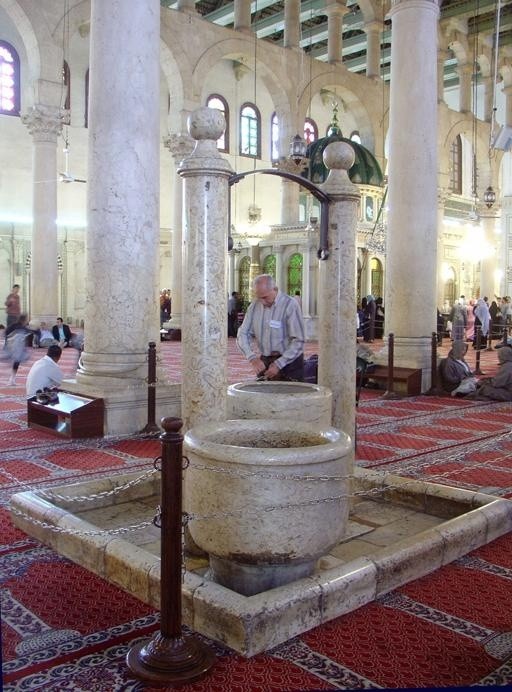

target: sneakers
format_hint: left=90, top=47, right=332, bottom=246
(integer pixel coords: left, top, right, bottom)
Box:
left=43, top=386, right=58, bottom=402
left=35, top=389, right=49, bottom=405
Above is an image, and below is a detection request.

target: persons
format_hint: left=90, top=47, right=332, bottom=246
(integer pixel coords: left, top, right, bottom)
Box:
left=228, top=292, right=238, bottom=337
left=5, top=285, right=71, bottom=401
left=293, top=291, right=301, bottom=305
left=236, top=274, right=306, bottom=382
left=357, top=294, right=512, bottom=401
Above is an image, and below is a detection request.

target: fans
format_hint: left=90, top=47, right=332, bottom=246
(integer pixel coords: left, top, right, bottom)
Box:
left=32, top=147, right=87, bottom=185
left=459, top=192, right=500, bottom=222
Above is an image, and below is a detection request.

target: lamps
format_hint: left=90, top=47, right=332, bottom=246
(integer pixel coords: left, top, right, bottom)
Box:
left=483, top=150, right=496, bottom=209
left=289, top=0, right=307, bottom=166
